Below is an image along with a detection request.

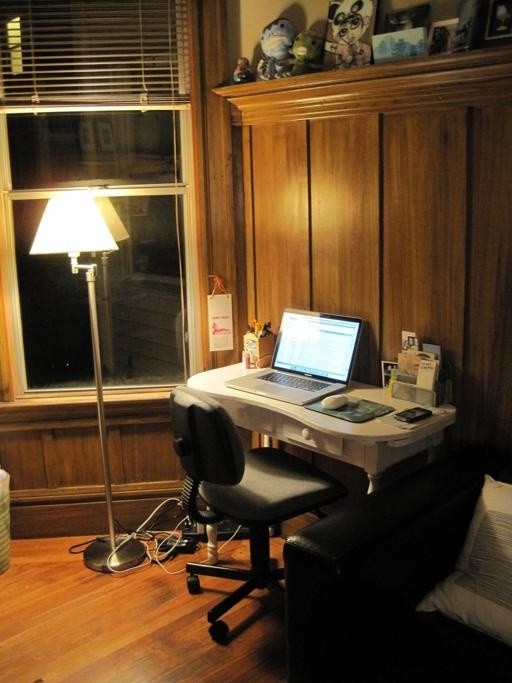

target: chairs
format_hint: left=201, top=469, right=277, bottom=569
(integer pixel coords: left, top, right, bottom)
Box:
left=169, top=395, right=344, bottom=645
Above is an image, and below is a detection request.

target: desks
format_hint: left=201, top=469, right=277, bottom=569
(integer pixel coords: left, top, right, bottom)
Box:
left=172, top=362, right=457, bottom=564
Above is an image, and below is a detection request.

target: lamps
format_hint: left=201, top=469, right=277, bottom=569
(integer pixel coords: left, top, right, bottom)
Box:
left=29, top=193, right=146, bottom=572
left=91, top=197, right=131, bottom=380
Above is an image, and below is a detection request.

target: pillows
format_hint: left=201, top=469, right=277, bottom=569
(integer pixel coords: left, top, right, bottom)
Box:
left=415, top=474, right=512, bottom=646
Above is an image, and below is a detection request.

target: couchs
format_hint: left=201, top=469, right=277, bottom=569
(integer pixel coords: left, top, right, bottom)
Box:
left=283, top=440, right=512, bottom=683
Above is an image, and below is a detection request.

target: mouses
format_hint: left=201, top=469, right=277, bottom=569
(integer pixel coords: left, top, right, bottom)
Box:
left=321, top=395, right=348, bottom=410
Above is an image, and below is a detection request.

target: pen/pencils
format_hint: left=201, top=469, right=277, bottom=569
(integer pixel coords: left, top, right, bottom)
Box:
left=247, top=322, right=271, bottom=338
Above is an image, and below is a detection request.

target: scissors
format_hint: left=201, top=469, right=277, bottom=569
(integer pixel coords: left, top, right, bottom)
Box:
left=252, top=320, right=261, bottom=335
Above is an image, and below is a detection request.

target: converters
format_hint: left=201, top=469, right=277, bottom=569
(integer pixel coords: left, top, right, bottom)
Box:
left=157, top=537, right=195, bottom=552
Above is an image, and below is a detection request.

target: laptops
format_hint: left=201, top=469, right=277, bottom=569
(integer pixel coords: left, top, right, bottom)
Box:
left=223, top=308, right=364, bottom=406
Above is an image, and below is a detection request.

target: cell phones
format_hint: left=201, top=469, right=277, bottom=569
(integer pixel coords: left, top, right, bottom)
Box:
left=394, top=407, right=432, bottom=423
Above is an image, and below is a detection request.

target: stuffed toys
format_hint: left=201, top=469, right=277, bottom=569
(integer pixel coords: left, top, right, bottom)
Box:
left=260, top=19, right=292, bottom=79
left=277, top=34, right=322, bottom=75
